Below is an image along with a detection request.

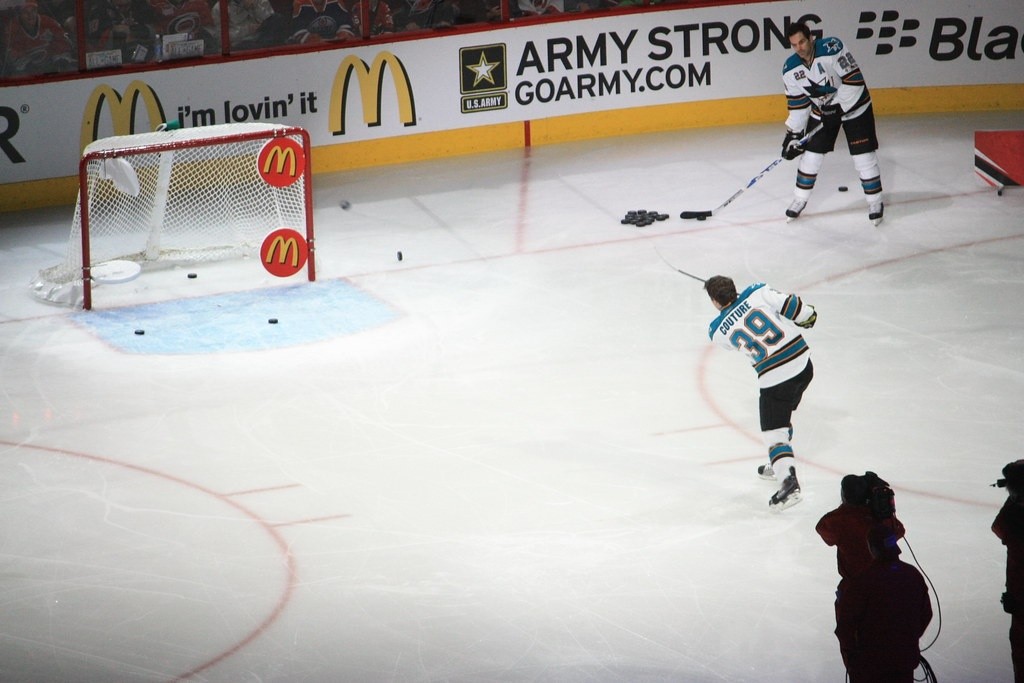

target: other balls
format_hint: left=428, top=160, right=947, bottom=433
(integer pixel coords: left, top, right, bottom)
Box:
left=135, top=330, right=144, bottom=335
left=397, top=252, right=403, bottom=261
left=188, top=273, right=197, bottom=278
left=697, top=216, right=706, bottom=220
left=621, top=209, right=670, bottom=227
left=268, top=318, right=278, bottom=324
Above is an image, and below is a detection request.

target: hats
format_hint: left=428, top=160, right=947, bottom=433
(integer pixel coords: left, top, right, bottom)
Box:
left=869, top=526, right=897, bottom=551
left=843, top=476, right=869, bottom=496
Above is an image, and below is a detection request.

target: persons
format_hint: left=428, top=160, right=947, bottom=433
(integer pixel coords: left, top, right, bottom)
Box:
left=782, top=23, right=884, bottom=226
left=991, top=459, right=1024, bottom=683
left=705, top=275, right=817, bottom=512
left=0, top=0, right=74, bottom=77
left=40, top=0, right=665, bottom=72
left=835, top=516, right=933, bottom=683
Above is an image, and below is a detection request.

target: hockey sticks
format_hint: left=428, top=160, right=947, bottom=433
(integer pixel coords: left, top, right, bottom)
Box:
left=679, top=122, right=824, bottom=219
left=661, top=256, right=742, bottom=299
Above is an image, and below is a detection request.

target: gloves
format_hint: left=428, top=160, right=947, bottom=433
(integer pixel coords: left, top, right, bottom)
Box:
left=782, top=131, right=805, bottom=159
left=818, top=104, right=845, bottom=123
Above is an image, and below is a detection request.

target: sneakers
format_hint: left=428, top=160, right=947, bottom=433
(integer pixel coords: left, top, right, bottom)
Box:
left=868, top=202, right=885, bottom=222
left=770, top=473, right=805, bottom=512
left=784, top=197, right=807, bottom=217
left=757, top=463, right=775, bottom=480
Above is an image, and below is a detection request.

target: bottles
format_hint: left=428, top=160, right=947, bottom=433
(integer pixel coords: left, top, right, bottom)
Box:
left=154, top=34, right=162, bottom=61
